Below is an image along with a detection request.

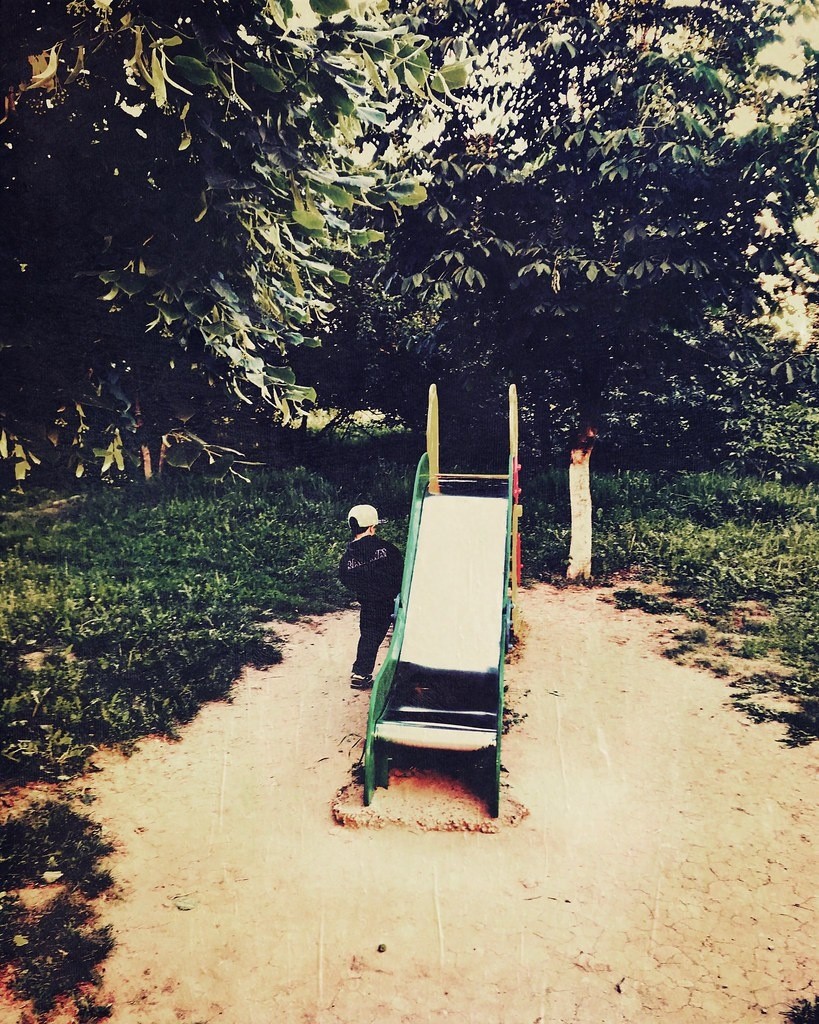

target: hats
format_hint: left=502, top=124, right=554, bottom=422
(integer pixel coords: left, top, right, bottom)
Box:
left=347, top=505, right=389, bottom=526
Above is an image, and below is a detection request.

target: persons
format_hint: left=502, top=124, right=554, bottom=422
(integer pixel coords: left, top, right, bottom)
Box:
left=339, top=503, right=406, bottom=689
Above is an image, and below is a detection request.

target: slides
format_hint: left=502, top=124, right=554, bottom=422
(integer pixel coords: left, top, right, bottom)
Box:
left=361, top=452, right=515, bottom=819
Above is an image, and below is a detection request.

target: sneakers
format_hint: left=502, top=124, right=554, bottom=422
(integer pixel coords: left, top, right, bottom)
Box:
left=350, top=675, right=374, bottom=689
